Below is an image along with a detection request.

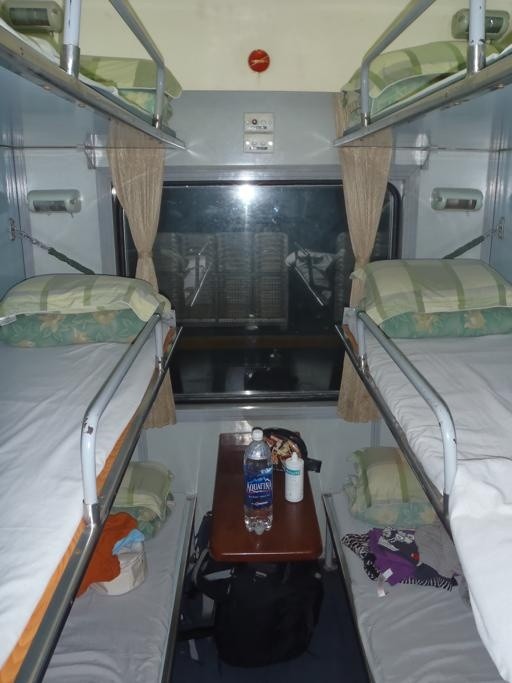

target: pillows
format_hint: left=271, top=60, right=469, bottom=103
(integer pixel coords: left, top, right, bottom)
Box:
left=75, top=54, right=182, bottom=100
left=339, top=40, right=499, bottom=108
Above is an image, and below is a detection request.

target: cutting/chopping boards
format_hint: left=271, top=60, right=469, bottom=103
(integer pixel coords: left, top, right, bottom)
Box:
left=262, top=427, right=321, bottom=473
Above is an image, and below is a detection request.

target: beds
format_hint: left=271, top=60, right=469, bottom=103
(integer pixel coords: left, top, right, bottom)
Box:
left=0, top=0, right=186, bottom=150
left=181, top=239, right=213, bottom=308
left=287, top=240, right=336, bottom=314
left=333, top=0, right=512, bottom=152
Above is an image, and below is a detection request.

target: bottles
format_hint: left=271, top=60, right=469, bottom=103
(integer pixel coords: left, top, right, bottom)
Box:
left=242, top=428, right=275, bottom=535
left=285, top=452, right=305, bottom=502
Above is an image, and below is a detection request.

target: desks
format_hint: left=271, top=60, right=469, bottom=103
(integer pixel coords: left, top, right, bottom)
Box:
left=207, top=432, right=323, bottom=561
